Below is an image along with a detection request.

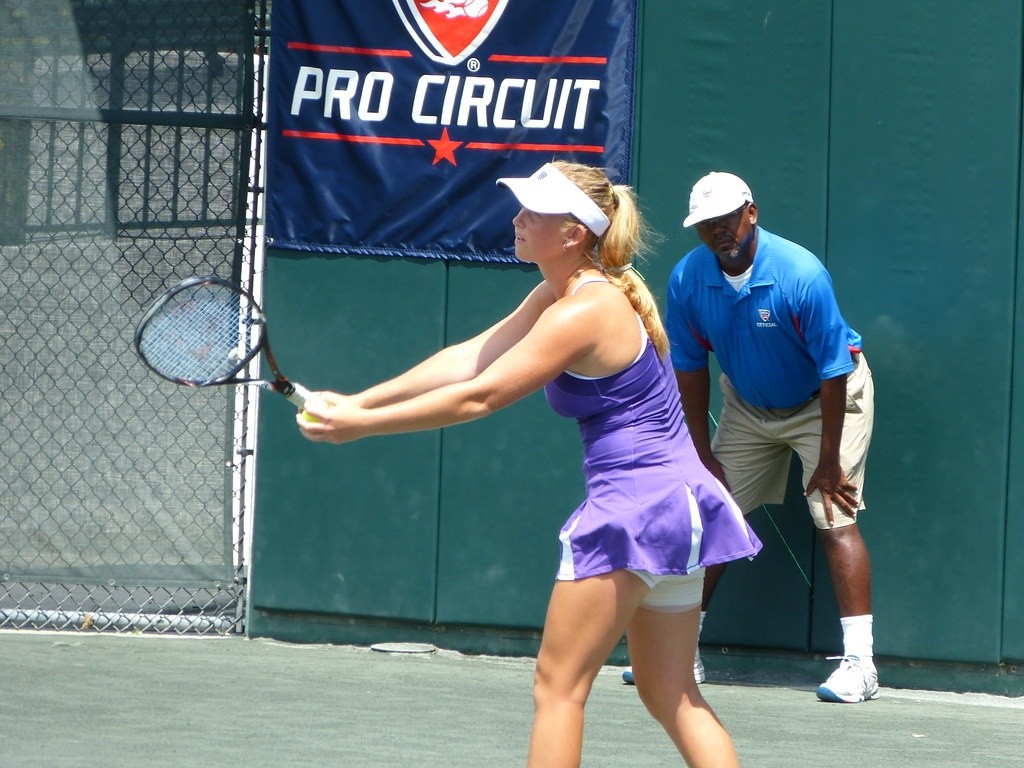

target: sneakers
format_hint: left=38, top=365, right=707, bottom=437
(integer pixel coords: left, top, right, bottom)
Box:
left=816, top=655, right=880, bottom=702
left=623, top=656, right=705, bottom=684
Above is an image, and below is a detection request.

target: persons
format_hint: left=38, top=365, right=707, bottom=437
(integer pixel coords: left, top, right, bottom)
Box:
left=621, top=170, right=883, bottom=704
left=294, top=158, right=763, bottom=768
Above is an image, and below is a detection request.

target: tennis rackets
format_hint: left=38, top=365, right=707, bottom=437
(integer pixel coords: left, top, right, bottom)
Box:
left=132, top=274, right=338, bottom=411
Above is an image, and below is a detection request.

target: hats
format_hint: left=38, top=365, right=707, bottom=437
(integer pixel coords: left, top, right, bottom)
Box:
left=683, top=172, right=753, bottom=236
left=496, top=162, right=610, bottom=237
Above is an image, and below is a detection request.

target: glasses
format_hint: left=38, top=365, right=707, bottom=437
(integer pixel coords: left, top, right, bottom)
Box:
left=695, top=203, right=747, bottom=231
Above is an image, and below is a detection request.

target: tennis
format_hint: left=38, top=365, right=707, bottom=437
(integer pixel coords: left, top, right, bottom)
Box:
left=302, top=408, right=323, bottom=423
